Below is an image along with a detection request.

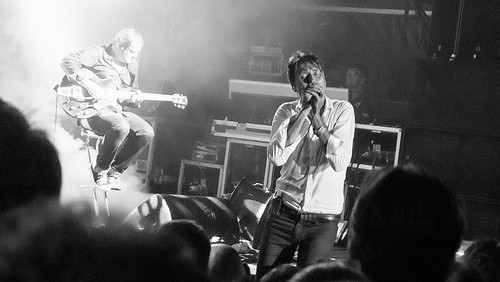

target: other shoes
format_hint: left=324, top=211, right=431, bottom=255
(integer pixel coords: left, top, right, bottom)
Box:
left=107, top=172, right=122, bottom=191
left=92, top=169, right=111, bottom=191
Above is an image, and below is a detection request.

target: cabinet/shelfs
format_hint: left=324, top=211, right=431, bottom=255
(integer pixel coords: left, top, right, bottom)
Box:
left=340, top=123, right=404, bottom=222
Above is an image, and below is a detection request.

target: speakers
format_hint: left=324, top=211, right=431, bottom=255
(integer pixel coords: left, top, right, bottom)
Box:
left=123, top=194, right=241, bottom=248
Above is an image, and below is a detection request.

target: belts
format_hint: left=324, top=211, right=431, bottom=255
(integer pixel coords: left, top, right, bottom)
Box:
left=283, top=205, right=341, bottom=222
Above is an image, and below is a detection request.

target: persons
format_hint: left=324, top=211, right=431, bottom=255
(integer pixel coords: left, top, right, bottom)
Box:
left=346, top=64, right=379, bottom=124
left=346, top=162, right=500, bottom=282
left=254, top=50, right=355, bottom=282
left=59, top=27, right=154, bottom=192
left=0, top=97, right=358, bottom=282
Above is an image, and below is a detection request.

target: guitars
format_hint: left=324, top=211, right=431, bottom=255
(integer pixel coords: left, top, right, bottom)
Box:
left=58, top=67, right=188, bottom=119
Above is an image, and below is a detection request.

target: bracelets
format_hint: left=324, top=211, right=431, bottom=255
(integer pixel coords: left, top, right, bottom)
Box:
left=313, top=124, right=328, bottom=136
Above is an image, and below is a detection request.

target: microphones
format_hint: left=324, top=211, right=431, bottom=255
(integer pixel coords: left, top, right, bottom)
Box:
left=310, top=96, right=318, bottom=114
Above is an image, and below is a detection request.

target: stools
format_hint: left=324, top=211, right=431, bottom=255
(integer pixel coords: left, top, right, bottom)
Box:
left=65, top=125, right=113, bottom=229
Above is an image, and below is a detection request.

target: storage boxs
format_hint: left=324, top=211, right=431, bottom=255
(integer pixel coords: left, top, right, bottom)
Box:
left=219, top=138, right=270, bottom=198
left=176, top=158, right=225, bottom=196
